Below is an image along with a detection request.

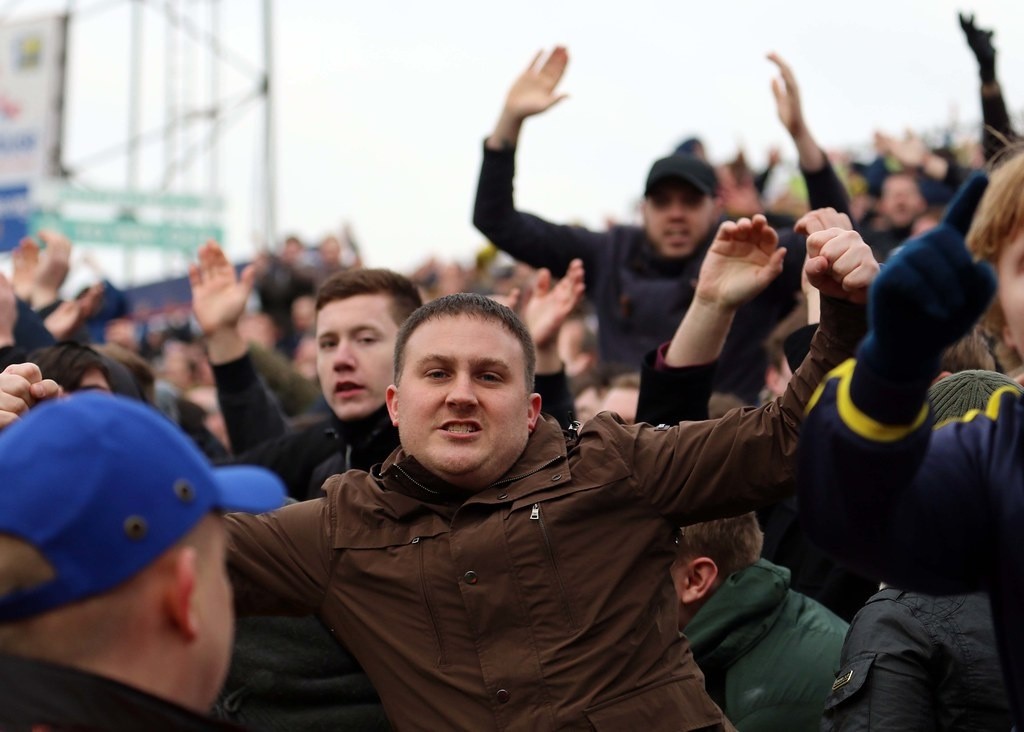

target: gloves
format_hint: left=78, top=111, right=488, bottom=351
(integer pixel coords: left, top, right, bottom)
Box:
left=852, top=172, right=996, bottom=419
left=959, top=14, right=997, bottom=86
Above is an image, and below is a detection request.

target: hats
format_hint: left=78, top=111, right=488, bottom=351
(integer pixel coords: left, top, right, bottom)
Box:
left=0, top=397, right=286, bottom=620
left=645, top=156, right=716, bottom=199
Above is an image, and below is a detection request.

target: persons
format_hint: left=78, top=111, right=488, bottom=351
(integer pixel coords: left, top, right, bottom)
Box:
left=0, top=392, right=292, bottom=731
left=468, top=44, right=857, bottom=376
left=821, top=368, right=1024, bottom=732
left=666, top=510, right=852, bottom=732
left=959, top=13, right=1024, bottom=169
left=1, top=224, right=652, bottom=439
left=187, top=232, right=590, bottom=501
left=757, top=147, right=1024, bottom=732
left=0, top=225, right=885, bottom=730
left=637, top=125, right=989, bottom=253
left=631, top=205, right=857, bottom=428
